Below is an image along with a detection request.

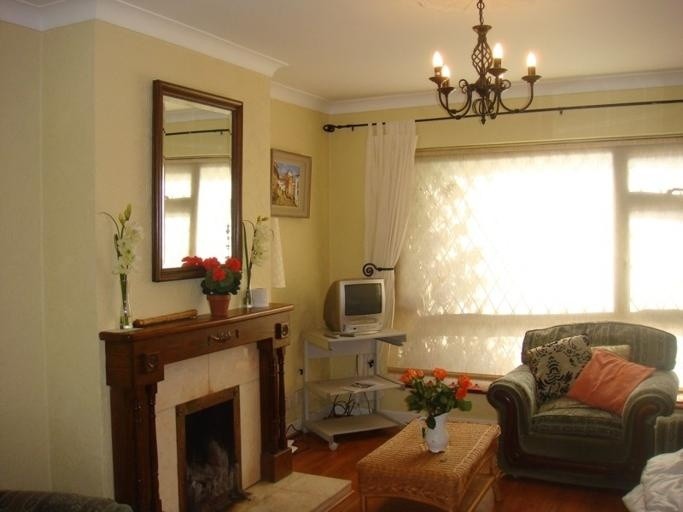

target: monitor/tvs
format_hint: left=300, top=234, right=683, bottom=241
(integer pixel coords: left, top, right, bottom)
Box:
left=323, top=279, right=386, bottom=333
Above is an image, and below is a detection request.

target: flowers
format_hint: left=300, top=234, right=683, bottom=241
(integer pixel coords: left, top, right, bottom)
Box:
left=96, top=202, right=144, bottom=325
left=180, top=256, right=204, bottom=268
left=239, top=215, right=274, bottom=306
left=200, top=257, right=242, bottom=296
left=396, top=366, right=481, bottom=438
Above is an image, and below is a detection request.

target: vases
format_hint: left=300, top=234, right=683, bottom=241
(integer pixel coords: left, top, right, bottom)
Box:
left=118, top=279, right=133, bottom=329
left=242, top=267, right=253, bottom=310
left=416, top=413, right=450, bottom=453
left=207, top=294, right=231, bottom=317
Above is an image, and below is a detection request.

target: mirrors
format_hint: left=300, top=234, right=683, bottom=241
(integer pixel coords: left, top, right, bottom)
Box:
left=151, top=77, right=243, bottom=283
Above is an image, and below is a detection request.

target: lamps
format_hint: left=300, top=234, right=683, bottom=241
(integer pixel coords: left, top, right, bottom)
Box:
left=427, top=0, right=542, bottom=126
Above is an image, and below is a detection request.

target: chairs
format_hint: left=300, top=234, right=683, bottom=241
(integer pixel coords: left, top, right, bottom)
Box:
left=487, top=321, right=679, bottom=491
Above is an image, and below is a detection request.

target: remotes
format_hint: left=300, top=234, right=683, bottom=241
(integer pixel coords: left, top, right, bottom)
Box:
left=340, top=334, right=355, bottom=337
left=323, top=333, right=339, bottom=339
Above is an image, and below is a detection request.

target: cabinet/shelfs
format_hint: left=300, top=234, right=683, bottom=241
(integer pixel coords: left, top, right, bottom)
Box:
left=303, top=327, right=407, bottom=451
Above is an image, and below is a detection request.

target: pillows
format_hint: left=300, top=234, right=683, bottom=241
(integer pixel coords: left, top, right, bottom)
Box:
left=523, top=334, right=657, bottom=418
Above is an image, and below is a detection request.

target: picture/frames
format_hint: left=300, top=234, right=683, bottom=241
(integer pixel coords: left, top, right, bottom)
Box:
left=269, top=147, right=313, bottom=220
